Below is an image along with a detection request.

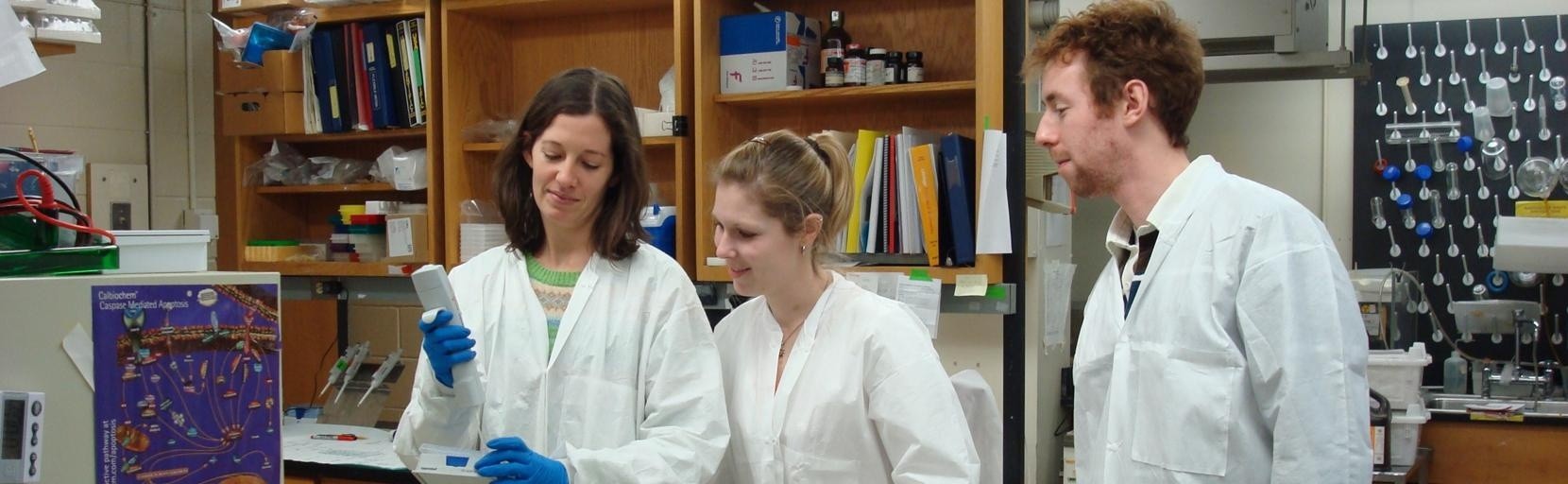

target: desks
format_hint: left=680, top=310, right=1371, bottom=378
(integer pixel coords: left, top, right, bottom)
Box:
left=281, top=413, right=424, bottom=483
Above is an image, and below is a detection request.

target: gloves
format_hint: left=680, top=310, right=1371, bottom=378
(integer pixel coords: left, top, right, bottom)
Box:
left=472, top=435, right=571, bottom=484
left=416, top=306, right=478, bottom=390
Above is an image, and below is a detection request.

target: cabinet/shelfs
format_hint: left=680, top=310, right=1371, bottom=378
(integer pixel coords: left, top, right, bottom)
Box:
left=698, top=6, right=1013, bottom=284
left=442, top=0, right=691, bottom=278
left=1423, top=406, right=1567, bottom=484
left=213, top=3, right=440, bottom=281
left=15, top=2, right=102, bottom=46
left=1371, top=445, right=1432, bottom=484
left=1, top=271, right=287, bottom=482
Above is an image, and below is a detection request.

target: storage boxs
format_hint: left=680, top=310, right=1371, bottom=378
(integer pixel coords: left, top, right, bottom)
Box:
left=222, top=89, right=306, bottom=138
left=0, top=147, right=88, bottom=246
left=383, top=212, right=429, bottom=255
left=719, top=10, right=826, bottom=91
left=102, top=230, right=212, bottom=273
left=219, top=45, right=306, bottom=94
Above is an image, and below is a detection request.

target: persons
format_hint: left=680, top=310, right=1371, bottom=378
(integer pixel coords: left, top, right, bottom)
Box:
left=1031, top=1, right=1374, bottom=484
left=387, top=57, right=733, bottom=484
left=711, top=128, right=979, bottom=483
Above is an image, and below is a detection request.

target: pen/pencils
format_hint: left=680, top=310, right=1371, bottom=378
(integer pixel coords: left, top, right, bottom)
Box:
left=310, top=434, right=357, bottom=441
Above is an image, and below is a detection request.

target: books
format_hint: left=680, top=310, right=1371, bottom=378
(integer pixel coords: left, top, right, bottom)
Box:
left=809, top=125, right=975, bottom=268
left=303, top=18, right=427, bottom=135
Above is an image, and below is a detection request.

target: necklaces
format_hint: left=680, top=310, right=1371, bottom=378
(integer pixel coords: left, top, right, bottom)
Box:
left=766, top=283, right=832, bottom=359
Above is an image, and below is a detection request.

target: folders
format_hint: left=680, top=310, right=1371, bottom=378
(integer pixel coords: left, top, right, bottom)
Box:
left=312, top=18, right=428, bottom=135
left=909, top=133, right=977, bottom=267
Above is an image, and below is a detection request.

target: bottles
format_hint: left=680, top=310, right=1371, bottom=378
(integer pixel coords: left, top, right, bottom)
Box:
left=1550, top=74, right=1566, bottom=110
left=1443, top=349, right=1467, bottom=393
left=817, top=8, right=924, bottom=87
left=1367, top=134, right=1461, bottom=230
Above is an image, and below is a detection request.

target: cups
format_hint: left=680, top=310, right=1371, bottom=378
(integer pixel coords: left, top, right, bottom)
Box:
left=1473, top=107, right=1496, bottom=141
left=1479, top=138, right=1511, bottom=180
left=1484, top=78, right=1513, bottom=117
